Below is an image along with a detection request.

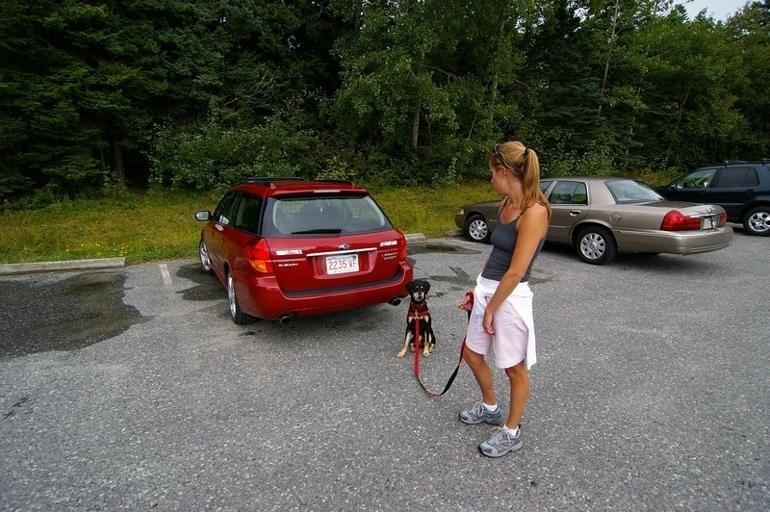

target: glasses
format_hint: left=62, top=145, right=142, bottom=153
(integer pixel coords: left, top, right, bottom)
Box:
left=493, top=144, right=500, bottom=157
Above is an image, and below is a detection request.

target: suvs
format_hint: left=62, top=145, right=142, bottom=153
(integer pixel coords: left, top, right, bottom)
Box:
left=653, top=159, right=770, bottom=237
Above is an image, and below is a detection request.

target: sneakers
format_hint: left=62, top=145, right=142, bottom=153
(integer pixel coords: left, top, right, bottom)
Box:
left=478, top=424, right=523, bottom=458
left=459, top=400, right=504, bottom=425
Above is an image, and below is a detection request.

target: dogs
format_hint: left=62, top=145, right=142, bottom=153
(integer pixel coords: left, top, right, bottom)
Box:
left=396, top=279, right=437, bottom=357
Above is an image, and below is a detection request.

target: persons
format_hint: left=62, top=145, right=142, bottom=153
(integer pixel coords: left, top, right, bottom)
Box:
left=454, top=140, right=551, bottom=460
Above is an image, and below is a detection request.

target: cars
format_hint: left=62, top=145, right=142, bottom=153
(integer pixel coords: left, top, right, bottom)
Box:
left=454, top=175, right=735, bottom=266
left=193, top=177, right=414, bottom=326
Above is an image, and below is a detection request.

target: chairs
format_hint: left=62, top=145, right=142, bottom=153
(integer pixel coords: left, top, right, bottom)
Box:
left=555, top=188, right=573, bottom=203
left=240, top=203, right=345, bottom=231
